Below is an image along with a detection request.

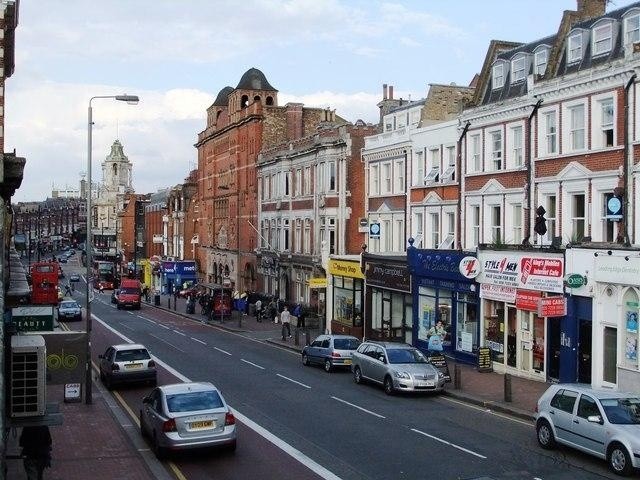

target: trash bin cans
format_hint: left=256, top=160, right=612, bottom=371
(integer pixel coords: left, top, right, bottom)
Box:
left=187, top=304, right=195, bottom=314
left=155, top=295, right=160, bottom=306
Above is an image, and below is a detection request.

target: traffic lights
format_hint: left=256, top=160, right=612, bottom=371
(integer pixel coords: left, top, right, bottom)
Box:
left=129, top=269, right=133, bottom=275
left=116, top=253, right=121, bottom=266
left=94, top=241, right=107, bottom=247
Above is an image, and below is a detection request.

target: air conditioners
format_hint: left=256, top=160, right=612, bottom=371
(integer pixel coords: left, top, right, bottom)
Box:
left=7, top=334, right=47, bottom=418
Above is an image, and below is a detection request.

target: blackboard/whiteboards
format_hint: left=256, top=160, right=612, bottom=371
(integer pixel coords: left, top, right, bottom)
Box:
left=479, top=348, right=492, bottom=368
left=428, top=357, right=451, bottom=384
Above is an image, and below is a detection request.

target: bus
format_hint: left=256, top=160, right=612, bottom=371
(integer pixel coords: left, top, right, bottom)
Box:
left=93, top=260, right=115, bottom=289
left=32, top=263, right=58, bottom=305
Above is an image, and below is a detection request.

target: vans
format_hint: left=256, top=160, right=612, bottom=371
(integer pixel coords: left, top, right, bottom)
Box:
left=117, top=280, right=141, bottom=310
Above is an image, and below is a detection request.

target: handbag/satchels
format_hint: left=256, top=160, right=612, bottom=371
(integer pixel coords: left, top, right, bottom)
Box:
left=428, top=335, right=443, bottom=351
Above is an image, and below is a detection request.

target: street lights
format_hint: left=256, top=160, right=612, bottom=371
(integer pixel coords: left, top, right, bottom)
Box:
left=217, top=184, right=242, bottom=328
left=85, top=93, right=141, bottom=404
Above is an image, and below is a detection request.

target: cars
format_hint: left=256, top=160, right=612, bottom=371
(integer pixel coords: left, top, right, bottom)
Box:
left=10, top=243, right=85, bottom=282
left=138, top=381, right=237, bottom=455
left=535, top=383, right=640, bottom=476
left=111, top=288, right=119, bottom=304
left=58, top=286, right=63, bottom=302
left=56, top=300, right=82, bottom=322
left=180, top=284, right=199, bottom=300
left=301, top=334, right=445, bottom=396
left=98, top=343, right=158, bottom=391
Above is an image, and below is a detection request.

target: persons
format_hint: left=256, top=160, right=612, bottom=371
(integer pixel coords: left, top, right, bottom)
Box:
left=19, top=426, right=53, bottom=480
left=64, top=283, right=75, bottom=296
left=98, top=283, right=104, bottom=294
left=207, top=296, right=215, bottom=321
left=143, top=286, right=150, bottom=301
left=427, top=320, right=447, bottom=356
left=240, top=291, right=306, bottom=338
